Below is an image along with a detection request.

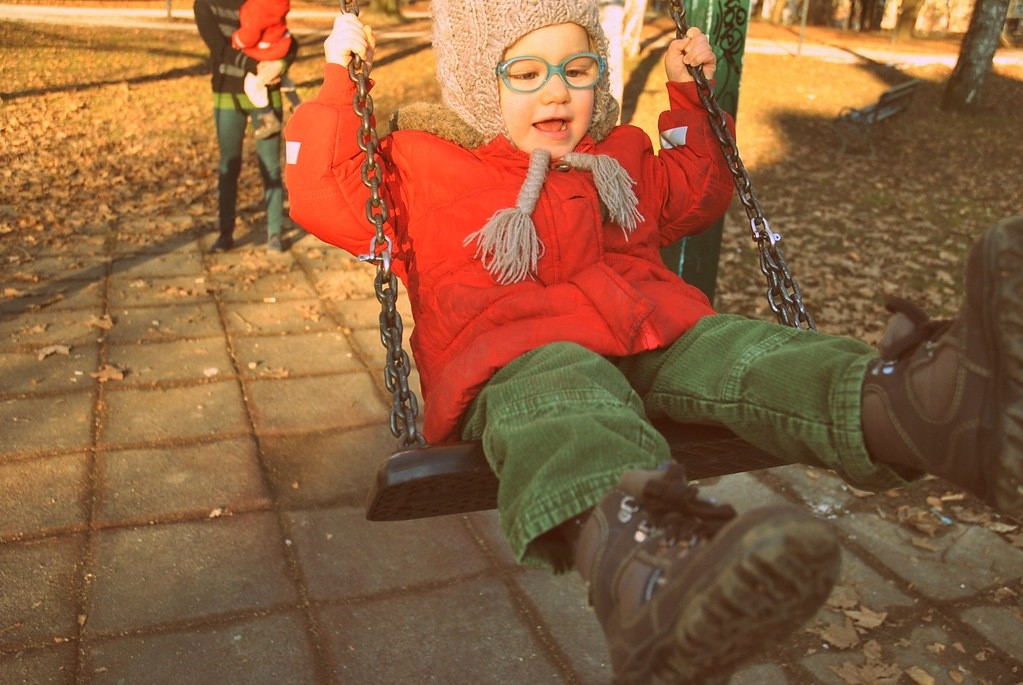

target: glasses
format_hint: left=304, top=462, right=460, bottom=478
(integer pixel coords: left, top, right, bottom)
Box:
left=496, top=52, right=606, bottom=93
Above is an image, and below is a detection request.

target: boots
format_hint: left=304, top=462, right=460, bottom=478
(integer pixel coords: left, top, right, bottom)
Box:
left=860, top=217, right=1023, bottom=522
left=570, top=456, right=841, bottom=685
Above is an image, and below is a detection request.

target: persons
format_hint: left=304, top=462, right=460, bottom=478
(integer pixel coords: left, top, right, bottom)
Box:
left=194, top=0, right=1023, bottom=685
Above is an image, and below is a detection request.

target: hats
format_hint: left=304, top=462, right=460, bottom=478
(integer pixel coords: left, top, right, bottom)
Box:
left=430, top=0, right=644, bottom=286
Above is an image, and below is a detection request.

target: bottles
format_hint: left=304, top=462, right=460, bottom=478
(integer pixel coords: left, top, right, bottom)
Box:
left=244, top=71, right=269, bottom=107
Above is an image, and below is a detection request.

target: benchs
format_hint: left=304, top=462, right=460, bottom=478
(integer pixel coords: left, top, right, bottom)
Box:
left=835, top=78, right=921, bottom=146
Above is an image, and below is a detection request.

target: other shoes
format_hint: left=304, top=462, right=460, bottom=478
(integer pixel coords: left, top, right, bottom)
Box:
left=212, top=234, right=233, bottom=253
left=254, top=110, right=281, bottom=138
left=267, top=233, right=282, bottom=253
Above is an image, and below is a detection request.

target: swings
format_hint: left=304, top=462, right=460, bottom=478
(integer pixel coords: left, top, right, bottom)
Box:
left=330, top=1, right=819, bottom=524
